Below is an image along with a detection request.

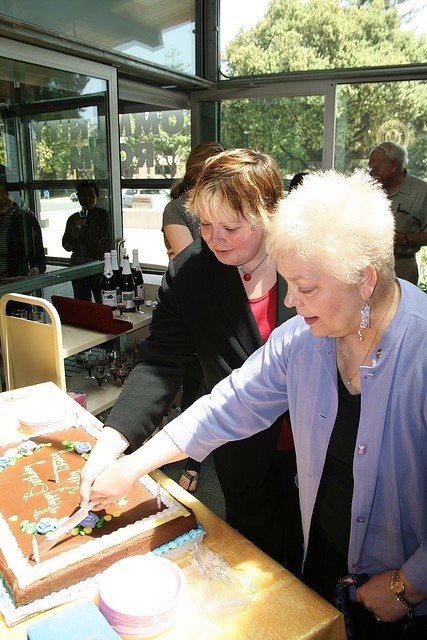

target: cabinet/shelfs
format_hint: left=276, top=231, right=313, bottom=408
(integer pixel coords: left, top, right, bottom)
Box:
left=0, top=263, right=185, bottom=447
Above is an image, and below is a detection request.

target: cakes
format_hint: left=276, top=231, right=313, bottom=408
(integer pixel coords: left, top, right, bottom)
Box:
left=1, top=421, right=207, bottom=627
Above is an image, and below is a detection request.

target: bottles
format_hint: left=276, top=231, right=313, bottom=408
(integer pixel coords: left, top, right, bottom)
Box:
left=129, top=249, right=144, bottom=297
left=99, top=252, right=116, bottom=311
left=121, top=255, right=135, bottom=312
left=118, top=247, right=126, bottom=301
left=110, top=250, right=122, bottom=307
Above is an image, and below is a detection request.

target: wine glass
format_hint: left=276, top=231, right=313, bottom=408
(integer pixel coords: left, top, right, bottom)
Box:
left=83, top=356, right=98, bottom=380
left=94, top=368, right=107, bottom=393
left=109, top=362, right=121, bottom=384
left=114, top=294, right=127, bottom=320
left=78, top=209, right=88, bottom=226
left=131, top=289, right=144, bottom=316
left=115, top=367, right=128, bottom=390
left=105, top=349, right=127, bottom=365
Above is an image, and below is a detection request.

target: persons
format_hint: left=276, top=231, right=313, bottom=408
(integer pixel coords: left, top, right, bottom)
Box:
left=87, top=169, right=426, bottom=639
left=0, top=164, right=48, bottom=322
left=161, top=141, right=227, bottom=261
left=365, top=142, right=426, bottom=286
left=62, top=182, right=110, bottom=305
left=74, top=144, right=303, bottom=578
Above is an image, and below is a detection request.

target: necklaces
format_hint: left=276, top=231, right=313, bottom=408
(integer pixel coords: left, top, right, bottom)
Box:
left=337, top=285, right=397, bottom=389
left=238, top=254, right=270, bottom=281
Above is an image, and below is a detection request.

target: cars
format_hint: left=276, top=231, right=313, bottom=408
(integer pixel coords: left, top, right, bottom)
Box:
left=123, top=189, right=169, bottom=209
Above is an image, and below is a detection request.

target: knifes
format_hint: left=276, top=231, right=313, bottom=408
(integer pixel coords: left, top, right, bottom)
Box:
left=28, top=498, right=100, bottom=562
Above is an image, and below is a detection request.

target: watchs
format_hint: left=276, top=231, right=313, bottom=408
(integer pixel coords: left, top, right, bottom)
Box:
left=387, top=568, right=414, bottom=611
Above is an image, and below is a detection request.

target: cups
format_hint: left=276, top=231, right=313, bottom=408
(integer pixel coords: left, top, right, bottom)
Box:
left=28, top=312, right=45, bottom=323
left=11, top=310, right=27, bottom=319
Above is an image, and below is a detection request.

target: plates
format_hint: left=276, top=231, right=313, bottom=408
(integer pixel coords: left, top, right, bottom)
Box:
left=98, top=554, right=188, bottom=639
left=18, top=410, right=78, bottom=434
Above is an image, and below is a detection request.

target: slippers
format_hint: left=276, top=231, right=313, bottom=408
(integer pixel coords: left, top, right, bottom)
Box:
left=178, top=470, right=200, bottom=494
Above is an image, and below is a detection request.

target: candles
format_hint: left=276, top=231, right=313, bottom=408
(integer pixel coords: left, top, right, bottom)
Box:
left=50, top=457, right=59, bottom=483
left=157, top=480, right=162, bottom=510
left=73, top=411, right=80, bottom=429
left=31, top=536, right=41, bottom=566
